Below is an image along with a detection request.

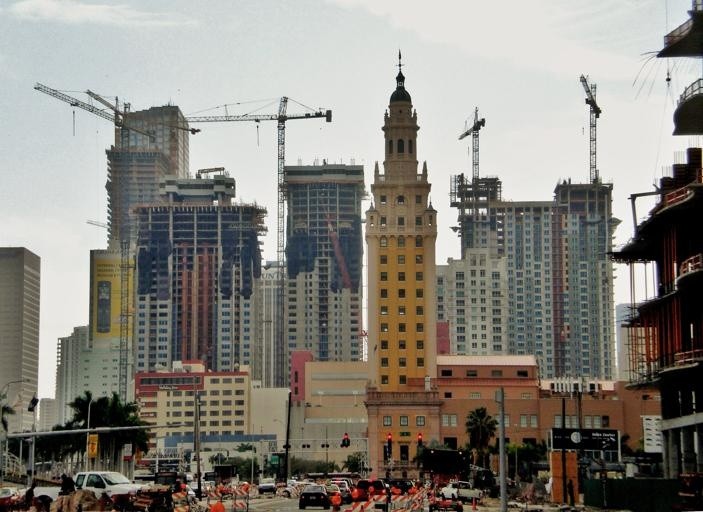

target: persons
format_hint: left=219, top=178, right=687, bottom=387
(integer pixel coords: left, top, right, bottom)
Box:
left=58, top=473, right=81, bottom=496
left=180, top=483, right=193, bottom=492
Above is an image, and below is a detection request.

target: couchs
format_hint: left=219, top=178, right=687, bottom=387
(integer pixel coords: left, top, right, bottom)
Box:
left=417, top=433, right=422, bottom=448
left=344, top=432, right=349, bottom=448
left=386, top=433, right=392, bottom=458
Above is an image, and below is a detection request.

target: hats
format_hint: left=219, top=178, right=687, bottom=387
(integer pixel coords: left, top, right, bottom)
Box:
left=186, top=473, right=485, bottom=512
left=0, top=486, right=18, bottom=499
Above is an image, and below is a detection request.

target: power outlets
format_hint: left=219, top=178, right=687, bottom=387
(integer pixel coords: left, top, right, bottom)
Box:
left=24, top=467, right=147, bottom=512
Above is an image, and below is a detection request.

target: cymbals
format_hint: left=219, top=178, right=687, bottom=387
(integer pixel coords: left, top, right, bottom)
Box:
left=83, top=86, right=200, bottom=407
left=33, top=78, right=158, bottom=238
left=178, top=90, right=335, bottom=381
left=576, top=71, right=605, bottom=185
left=456, top=105, right=485, bottom=216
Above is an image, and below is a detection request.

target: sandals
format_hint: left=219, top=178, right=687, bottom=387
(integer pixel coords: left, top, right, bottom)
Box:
left=133, top=474, right=155, bottom=487
left=154, top=471, right=188, bottom=495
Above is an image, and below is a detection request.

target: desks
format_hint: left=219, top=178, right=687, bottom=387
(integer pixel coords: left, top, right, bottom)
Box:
left=85, top=393, right=113, bottom=472
left=492, top=385, right=509, bottom=512
